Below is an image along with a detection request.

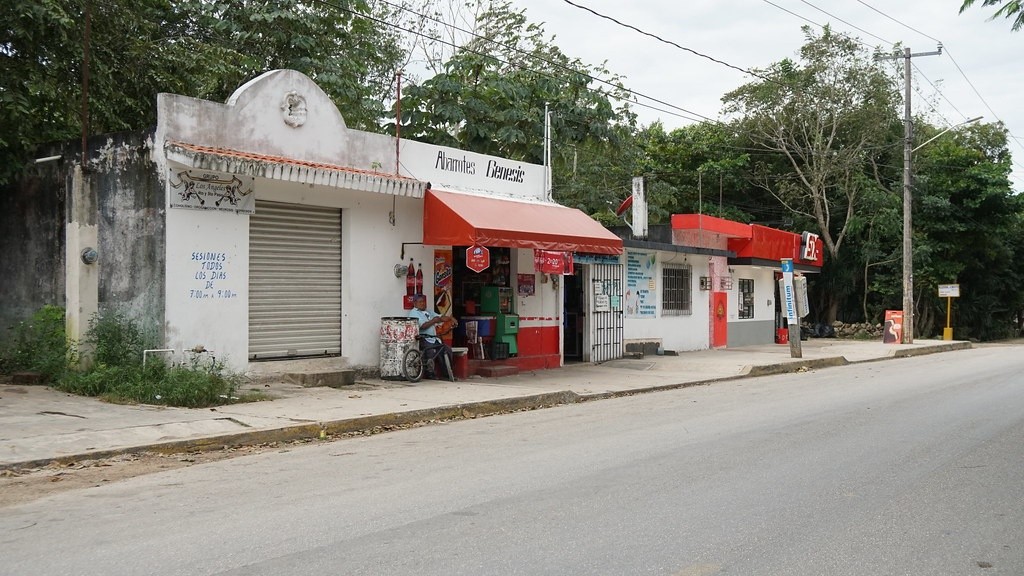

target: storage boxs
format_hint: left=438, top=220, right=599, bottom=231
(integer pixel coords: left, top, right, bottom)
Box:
left=480, top=273, right=493, bottom=281
left=485, top=342, right=510, bottom=359
left=459, top=316, right=497, bottom=336
left=462, top=282, right=494, bottom=307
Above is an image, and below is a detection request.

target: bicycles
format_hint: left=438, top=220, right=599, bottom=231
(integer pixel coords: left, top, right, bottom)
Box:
left=402, top=326, right=455, bottom=382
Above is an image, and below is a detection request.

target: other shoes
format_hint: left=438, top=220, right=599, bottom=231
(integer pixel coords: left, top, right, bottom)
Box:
left=424, top=370, right=441, bottom=380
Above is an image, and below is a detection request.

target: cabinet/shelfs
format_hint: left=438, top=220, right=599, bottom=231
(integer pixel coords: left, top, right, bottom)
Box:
left=565, top=312, right=581, bottom=357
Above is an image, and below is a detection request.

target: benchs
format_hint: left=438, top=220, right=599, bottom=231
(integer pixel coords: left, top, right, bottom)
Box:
left=421, top=347, right=469, bottom=378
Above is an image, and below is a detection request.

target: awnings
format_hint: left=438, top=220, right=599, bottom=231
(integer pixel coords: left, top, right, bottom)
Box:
left=423, top=189, right=623, bottom=255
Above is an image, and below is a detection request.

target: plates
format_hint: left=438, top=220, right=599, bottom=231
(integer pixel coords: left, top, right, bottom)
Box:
left=435, top=267, right=452, bottom=285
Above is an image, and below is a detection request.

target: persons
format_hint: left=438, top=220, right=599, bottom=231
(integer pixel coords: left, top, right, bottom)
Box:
left=409, top=295, right=457, bottom=375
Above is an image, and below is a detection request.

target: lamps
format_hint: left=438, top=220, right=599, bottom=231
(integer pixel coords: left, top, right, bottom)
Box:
left=394, top=264, right=409, bottom=277
left=82, top=247, right=99, bottom=264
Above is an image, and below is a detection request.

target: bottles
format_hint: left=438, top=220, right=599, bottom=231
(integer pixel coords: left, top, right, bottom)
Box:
left=416, top=263, right=423, bottom=295
left=540, top=275, right=547, bottom=285
left=406, top=258, right=415, bottom=295
left=545, top=283, right=545, bottom=285
left=551, top=274, right=559, bottom=291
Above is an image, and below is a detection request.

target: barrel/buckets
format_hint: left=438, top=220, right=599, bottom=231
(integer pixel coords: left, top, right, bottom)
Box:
left=466, top=301, right=475, bottom=316
left=452, top=347, right=469, bottom=378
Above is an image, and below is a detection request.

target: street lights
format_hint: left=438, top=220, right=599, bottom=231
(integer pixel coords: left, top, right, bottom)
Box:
left=903, top=116, right=984, bottom=344
